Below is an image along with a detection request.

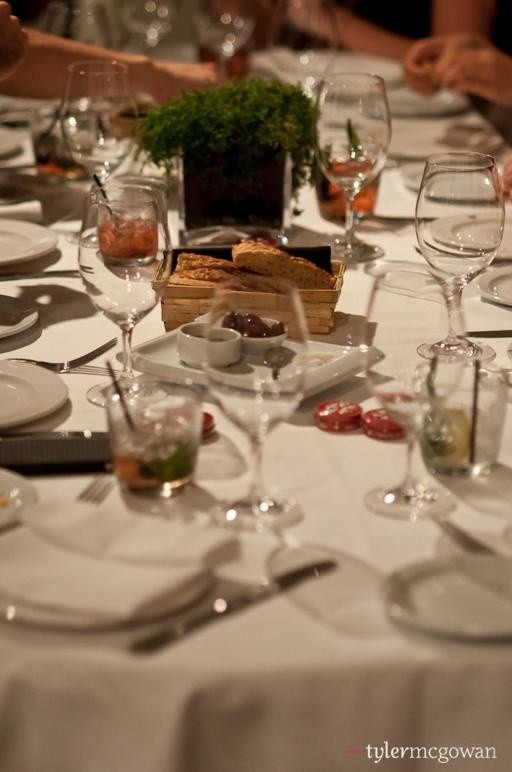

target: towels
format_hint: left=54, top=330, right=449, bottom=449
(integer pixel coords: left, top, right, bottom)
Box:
left=0, top=493, right=283, bottom=640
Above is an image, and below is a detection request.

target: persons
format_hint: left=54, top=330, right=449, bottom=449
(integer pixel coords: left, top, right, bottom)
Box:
left=0, top=1, right=511, bottom=198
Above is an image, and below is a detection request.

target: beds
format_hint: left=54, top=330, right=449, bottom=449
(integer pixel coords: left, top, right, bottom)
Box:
left=0, top=49, right=510, bottom=770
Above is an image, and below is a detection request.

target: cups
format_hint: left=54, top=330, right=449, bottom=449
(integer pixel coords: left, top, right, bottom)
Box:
left=420, top=348, right=504, bottom=482
left=315, top=141, right=383, bottom=225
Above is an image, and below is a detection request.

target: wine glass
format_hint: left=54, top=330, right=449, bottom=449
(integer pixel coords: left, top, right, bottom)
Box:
left=311, top=73, right=393, bottom=267
left=360, top=257, right=465, bottom=522
left=413, top=150, right=502, bottom=366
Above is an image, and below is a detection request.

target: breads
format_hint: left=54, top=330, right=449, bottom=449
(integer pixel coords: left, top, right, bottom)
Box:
left=158, top=237, right=338, bottom=336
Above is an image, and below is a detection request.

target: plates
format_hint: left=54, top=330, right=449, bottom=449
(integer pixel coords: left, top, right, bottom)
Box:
left=2, top=1, right=323, bottom=633
left=117, top=321, right=385, bottom=397
left=474, top=263, right=511, bottom=307
left=404, top=158, right=506, bottom=201
left=382, top=118, right=504, bottom=159
left=369, top=180, right=511, bottom=220
left=371, top=86, right=471, bottom=117
left=388, top=551, right=511, bottom=641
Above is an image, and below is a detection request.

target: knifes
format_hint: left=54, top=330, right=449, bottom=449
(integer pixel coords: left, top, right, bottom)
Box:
left=132, top=559, right=333, bottom=655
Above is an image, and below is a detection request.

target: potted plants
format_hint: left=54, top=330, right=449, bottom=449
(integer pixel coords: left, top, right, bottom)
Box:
left=135, top=76, right=320, bottom=237
